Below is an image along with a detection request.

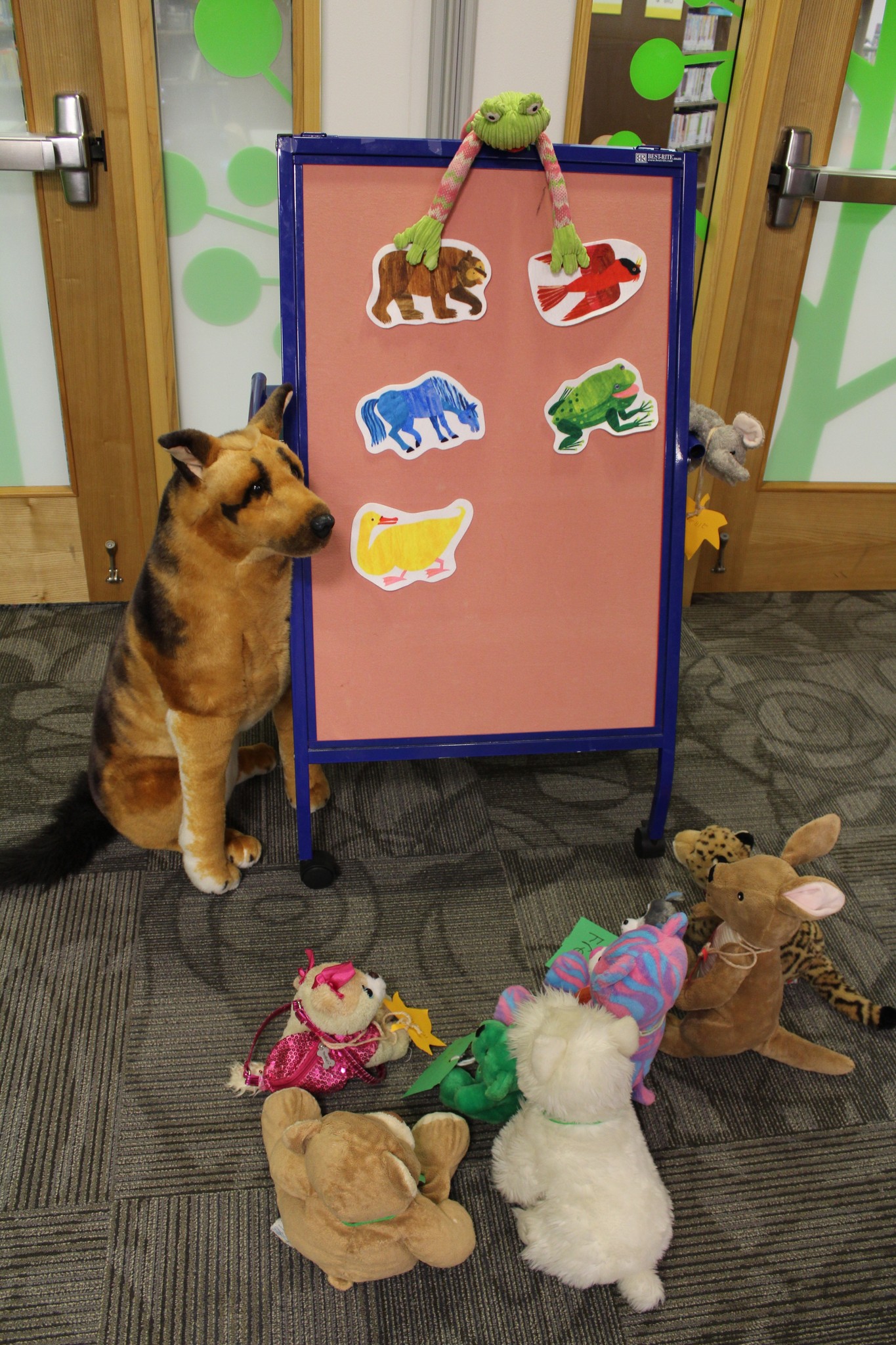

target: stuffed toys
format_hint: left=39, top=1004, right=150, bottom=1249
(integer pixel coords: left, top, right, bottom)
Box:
left=0, top=382, right=335, bottom=895
left=495, top=912, right=688, bottom=1105
left=261, top=1088, right=476, bottom=1291
left=440, top=1019, right=526, bottom=1123
left=657, top=814, right=855, bottom=1075
left=672, top=825, right=896, bottom=1029
left=490, top=990, right=674, bottom=1313
left=394, top=90, right=590, bottom=275
left=621, top=895, right=685, bottom=935
left=689, top=398, right=766, bottom=488
left=225, top=950, right=411, bottom=1095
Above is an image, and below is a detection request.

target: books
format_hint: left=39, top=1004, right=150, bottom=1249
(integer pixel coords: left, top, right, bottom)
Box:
left=668, top=110, right=716, bottom=149
left=681, top=12, right=718, bottom=50
left=675, top=65, right=717, bottom=103
